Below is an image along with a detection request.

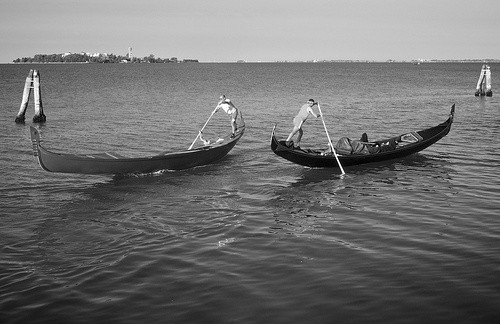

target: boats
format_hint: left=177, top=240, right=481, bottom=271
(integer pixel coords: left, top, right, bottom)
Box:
left=30, top=126, right=245, bottom=175
left=271, top=104, right=456, bottom=168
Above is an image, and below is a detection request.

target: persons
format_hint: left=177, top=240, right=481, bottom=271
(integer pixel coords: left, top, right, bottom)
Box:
left=285, top=99, right=321, bottom=148
left=211, top=94, right=239, bottom=140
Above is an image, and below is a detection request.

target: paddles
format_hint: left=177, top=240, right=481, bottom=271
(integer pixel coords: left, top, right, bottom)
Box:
left=316, top=101, right=346, bottom=175
left=187, top=101, right=223, bottom=151
